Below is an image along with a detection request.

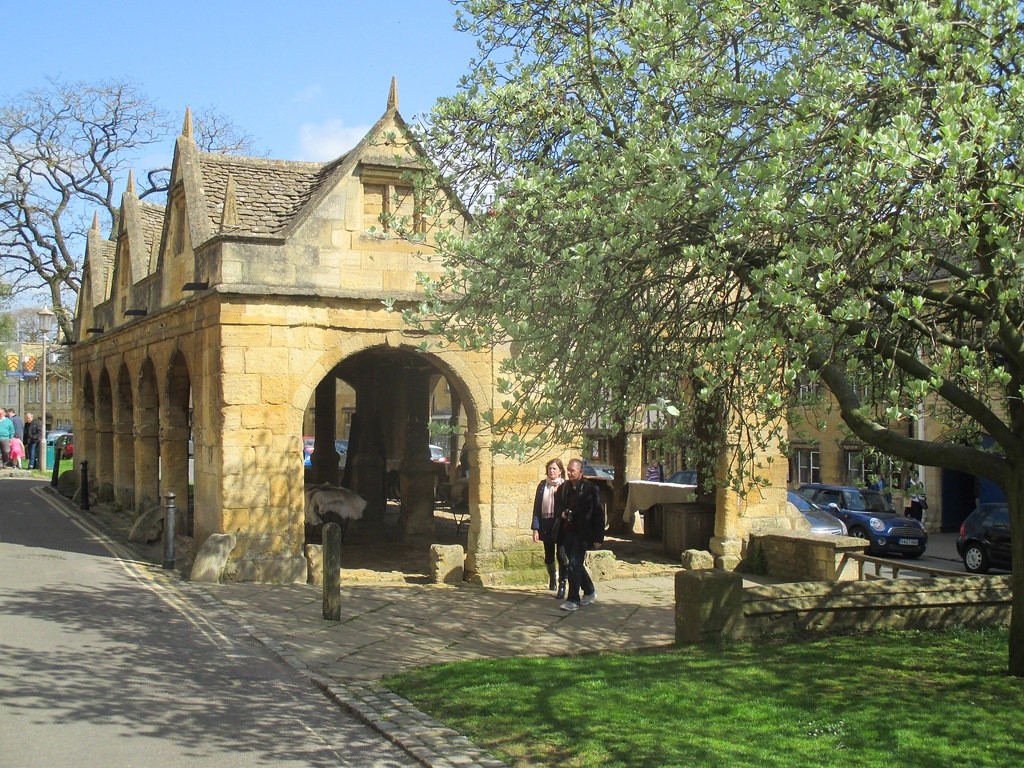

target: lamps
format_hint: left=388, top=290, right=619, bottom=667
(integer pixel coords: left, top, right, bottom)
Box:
left=61, top=341, right=76, bottom=345
left=124, top=310, right=147, bottom=316
left=87, top=326, right=104, bottom=334
left=179, top=282, right=218, bottom=291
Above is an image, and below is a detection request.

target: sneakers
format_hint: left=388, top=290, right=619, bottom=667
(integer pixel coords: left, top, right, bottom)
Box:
left=560, top=601, right=579, bottom=611
left=580, top=592, right=597, bottom=605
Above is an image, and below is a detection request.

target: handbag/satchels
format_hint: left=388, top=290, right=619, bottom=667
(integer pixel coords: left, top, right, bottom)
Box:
left=550, top=518, right=562, bottom=543
left=919, top=499, right=928, bottom=510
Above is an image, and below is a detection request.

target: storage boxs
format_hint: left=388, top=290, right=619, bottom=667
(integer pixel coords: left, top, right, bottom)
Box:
left=437, top=474, right=456, bottom=483
left=432, top=461, right=450, bottom=475
left=386, top=458, right=401, bottom=473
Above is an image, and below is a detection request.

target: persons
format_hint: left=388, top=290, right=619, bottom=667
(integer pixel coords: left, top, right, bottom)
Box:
left=460, top=430, right=470, bottom=479
left=554, top=459, right=605, bottom=610
left=866, top=466, right=883, bottom=492
left=9, top=433, right=25, bottom=469
left=6, top=409, right=25, bottom=442
left=531, top=458, right=566, bottom=598
left=24, top=413, right=41, bottom=470
left=645, top=460, right=659, bottom=481
left=0, top=408, right=14, bottom=469
left=905, top=470, right=926, bottom=521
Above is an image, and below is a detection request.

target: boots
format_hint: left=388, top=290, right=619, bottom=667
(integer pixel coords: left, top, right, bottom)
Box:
left=546, top=562, right=556, bottom=590
left=557, top=559, right=567, bottom=598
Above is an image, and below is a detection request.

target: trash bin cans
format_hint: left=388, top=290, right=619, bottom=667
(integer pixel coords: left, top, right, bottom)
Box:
left=46, top=438, right=55, bottom=470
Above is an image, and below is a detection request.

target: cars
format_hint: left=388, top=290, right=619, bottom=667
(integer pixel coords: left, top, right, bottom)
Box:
left=666, top=470, right=698, bottom=485
left=797, top=482, right=929, bottom=558
left=583, top=463, right=614, bottom=479
left=428, top=444, right=449, bottom=462
left=302, top=435, right=349, bottom=468
left=46, top=426, right=73, bottom=460
left=787, top=488, right=849, bottom=537
left=955, top=502, right=1012, bottom=574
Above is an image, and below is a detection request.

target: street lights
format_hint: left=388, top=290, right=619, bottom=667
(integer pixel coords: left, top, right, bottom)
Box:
left=36, top=303, right=55, bottom=472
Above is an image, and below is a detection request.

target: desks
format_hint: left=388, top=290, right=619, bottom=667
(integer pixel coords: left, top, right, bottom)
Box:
left=433, top=478, right=469, bottom=535
left=304, top=485, right=368, bottom=544
left=375, top=471, right=402, bottom=514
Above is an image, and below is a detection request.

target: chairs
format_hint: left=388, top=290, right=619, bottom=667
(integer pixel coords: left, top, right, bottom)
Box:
left=823, top=491, right=840, bottom=503
left=847, top=499, right=864, bottom=510
left=872, top=499, right=885, bottom=511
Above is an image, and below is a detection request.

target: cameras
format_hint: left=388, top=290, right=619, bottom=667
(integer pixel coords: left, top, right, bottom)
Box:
left=564, top=509, right=576, bottom=525
